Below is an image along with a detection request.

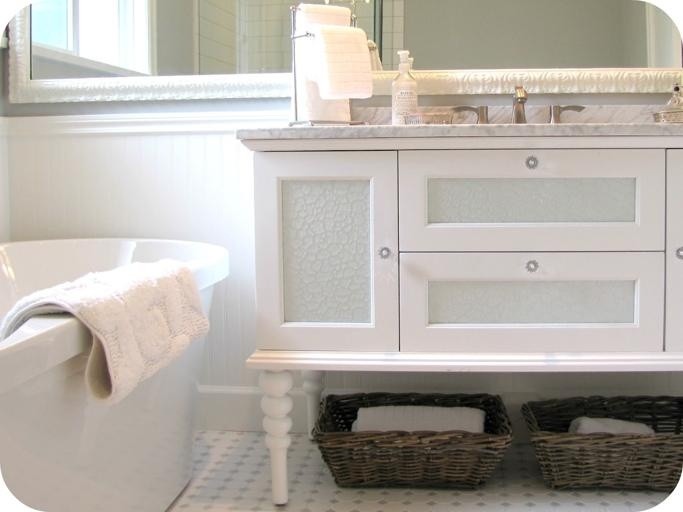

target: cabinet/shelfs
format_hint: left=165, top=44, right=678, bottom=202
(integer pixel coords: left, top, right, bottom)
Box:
left=395, top=137, right=663, bottom=358
left=661, top=136, right=682, bottom=357
left=242, top=137, right=400, bottom=351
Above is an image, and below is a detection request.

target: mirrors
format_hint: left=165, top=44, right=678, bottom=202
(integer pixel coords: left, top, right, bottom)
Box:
left=7, top=0, right=683, bottom=107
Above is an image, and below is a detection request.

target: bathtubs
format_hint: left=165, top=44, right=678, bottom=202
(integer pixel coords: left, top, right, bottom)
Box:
left=0, top=237, right=233, bottom=512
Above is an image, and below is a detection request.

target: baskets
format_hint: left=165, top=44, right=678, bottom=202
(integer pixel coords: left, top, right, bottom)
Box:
left=520, top=396, right=683, bottom=493
left=311, top=392, right=513, bottom=490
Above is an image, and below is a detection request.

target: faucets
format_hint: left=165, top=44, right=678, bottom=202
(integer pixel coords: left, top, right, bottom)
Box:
left=510, top=86, right=529, bottom=124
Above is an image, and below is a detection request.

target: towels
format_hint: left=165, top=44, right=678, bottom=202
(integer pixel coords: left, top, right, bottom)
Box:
left=0, top=259, right=212, bottom=405
left=350, top=404, right=487, bottom=436
left=566, top=414, right=653, bottom=438
